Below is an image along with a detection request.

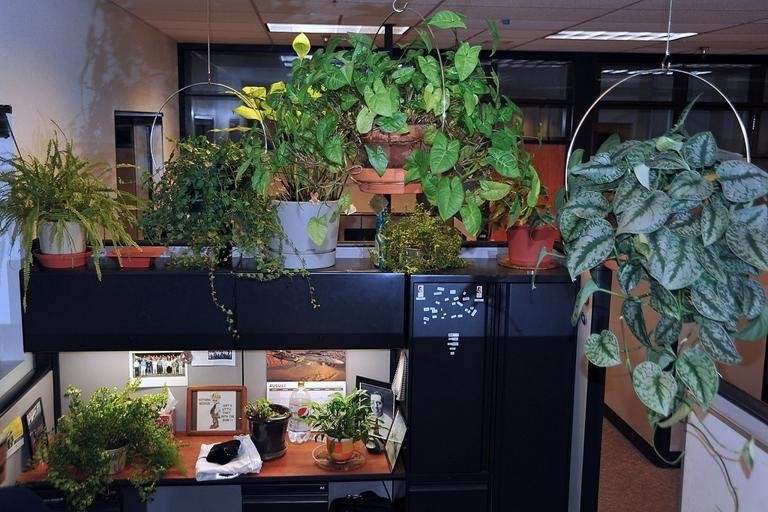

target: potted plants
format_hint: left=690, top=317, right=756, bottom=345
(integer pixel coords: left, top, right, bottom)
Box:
left=552, top=89, right=766, bottom=512
left=18, top=379, right=181, bottom=510
left=0, top=14, right=559, bottom=270
left=242, top=387, right=383, bottom=465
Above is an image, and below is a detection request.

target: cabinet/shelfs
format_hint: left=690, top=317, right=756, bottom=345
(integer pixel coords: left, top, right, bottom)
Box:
left=410, top=274, right=572, bottom=512
left=12, top=271, right=404, bottom=347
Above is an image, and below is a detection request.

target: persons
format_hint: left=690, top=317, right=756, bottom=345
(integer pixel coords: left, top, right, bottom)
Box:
left=364, top=391, right=392, bottom=438
left=208, top=350, right=232, bottom=359
left=418, top=287, right=422, bottom=292
left=134, top=354, right=186, bottom=378
left=477, top=286, right=481, bottom=295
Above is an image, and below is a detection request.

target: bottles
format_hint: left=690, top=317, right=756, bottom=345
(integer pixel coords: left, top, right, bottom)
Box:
left=288, top=380, right=311, bottom=445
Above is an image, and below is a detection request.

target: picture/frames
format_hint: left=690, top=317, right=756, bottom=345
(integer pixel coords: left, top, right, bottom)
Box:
left=354, top=375, right=398, bottom=444
left=185, top=385, right=249, bottom=436
